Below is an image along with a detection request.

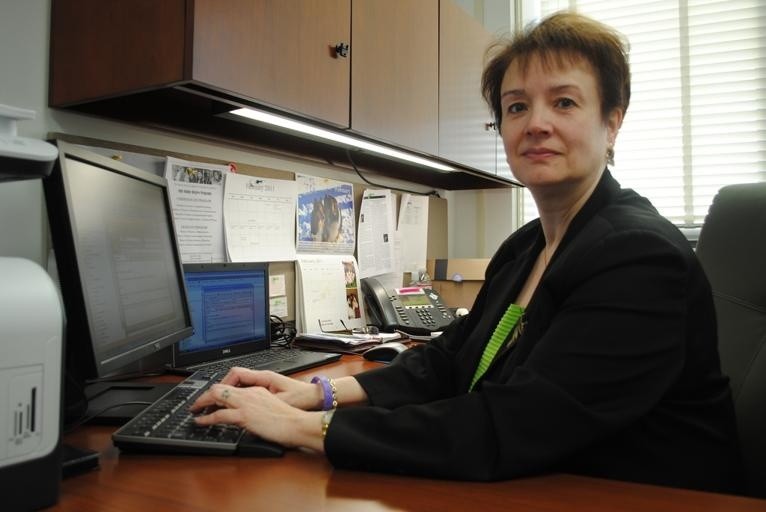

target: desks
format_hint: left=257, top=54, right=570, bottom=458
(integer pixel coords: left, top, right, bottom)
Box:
left=36, top=340, right=766, bottom=512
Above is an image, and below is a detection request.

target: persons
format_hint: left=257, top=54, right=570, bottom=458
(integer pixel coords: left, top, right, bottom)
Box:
left=190, top=12, right=740, bottom=496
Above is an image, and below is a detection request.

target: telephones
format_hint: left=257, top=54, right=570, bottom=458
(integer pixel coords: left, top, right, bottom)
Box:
left=360, top=277, right=455, bottom=333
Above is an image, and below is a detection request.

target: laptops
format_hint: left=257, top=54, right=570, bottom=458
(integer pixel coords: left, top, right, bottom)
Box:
left=172, top=262, right=341, bottom=382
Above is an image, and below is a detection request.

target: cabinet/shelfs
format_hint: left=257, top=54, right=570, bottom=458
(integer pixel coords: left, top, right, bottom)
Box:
left=438, top=0, right=525, bottom=192
left=48, top=0, right=438, bottom=189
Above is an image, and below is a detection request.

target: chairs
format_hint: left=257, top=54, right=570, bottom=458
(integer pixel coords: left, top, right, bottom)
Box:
left=695, top=182, right=766, bottom=499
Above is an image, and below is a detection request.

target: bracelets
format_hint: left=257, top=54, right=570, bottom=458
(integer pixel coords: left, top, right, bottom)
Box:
left=327, top=375, right=338, bottom=409
left=319, top=410, right=340, bottom=439
left=311, top=374, right=333, bottom=411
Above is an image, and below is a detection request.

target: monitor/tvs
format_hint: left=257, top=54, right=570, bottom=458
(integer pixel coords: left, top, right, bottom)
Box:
left=41, top=137, right=194, bottom=423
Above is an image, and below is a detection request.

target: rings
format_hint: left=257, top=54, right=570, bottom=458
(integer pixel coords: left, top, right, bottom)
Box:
left=219, top=386, right=235, bottom=406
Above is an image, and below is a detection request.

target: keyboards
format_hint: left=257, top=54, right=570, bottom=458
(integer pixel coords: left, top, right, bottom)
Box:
left=110, top=367, right=286, bottom=458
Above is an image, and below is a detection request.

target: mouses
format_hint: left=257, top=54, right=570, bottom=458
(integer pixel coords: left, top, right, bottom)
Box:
left=363, top=341, right=408, bottom=361
